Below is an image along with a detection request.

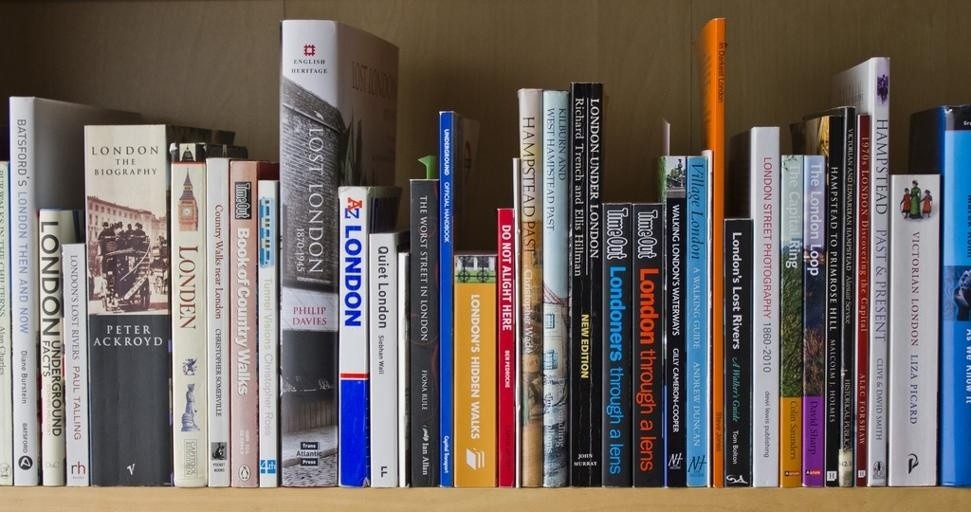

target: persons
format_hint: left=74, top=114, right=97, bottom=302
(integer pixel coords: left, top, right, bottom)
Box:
left=900, top=188, right=911, bottom=218
left=909, top=180, right=924, bottom=219
left=920, top=190, right=932, bottom=217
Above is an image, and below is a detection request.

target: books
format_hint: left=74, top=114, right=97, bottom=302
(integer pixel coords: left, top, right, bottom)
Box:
left=369, top=228, right=411, bottom=490
left=684, top=155, right=709, bottom=488
left=9, top=95, right=144, bottom=488
left=61, top=243, right=88, bottom=488
left=452, top=253, right=497, bottom=489
left=888, top=174, right=940, bottom=486
left=279, top=17, right=401, bottom=488
left=803, top=154, right=827, bottom=488
left=853, top=113, right=871, bottom=488
left=569, top=81, right=590, bottom=487
left=439, top=110, right=478, bottom=488
left=337, top=185, right=404, bottom=488
left=257, top=180, right=280, bottom=488
left=206, top=157, right=261, bottom=488
left=1, top=159, right=13, bottom=487
left=518, top=87, right=549, bottom=488
left=695, top=18, right=725, bottom=489
left=729, top=126, right=778, bottom=487
left=513, top=158, right=521, bottom=489
left=658, top=155, right=688, bottom=489
left=631, top=204, right=666, bottom=487
left=601, top=203, right=632, bottom=488
left=909, top=106, right=970, bottom=487
left=170, top=140, right=249, bottom=488
left=39, top=209, right=86, bottom=487
left=725, top=219, right=752, bottom=488
left=229, top=160, right=283, bottom=488
left=789, top=115, right=842, bottom=487
left=397, top=251, right=410, bottom=487
left=780, top=155, right=804, bottom=489
left=829, top=56, right=887, bottom=488
left=802, top=107, right=856, bottom=488
left=84, top=126, right=237, bottom=486
left=497, top=207, right=516, bottom=489
left=701, top=151, right=712, bottom=491
left=543, top=91, right=567, bottom=490
left=587, top=83, right=602, bottom=487
left=410, top=179, right=442, bottom=486
